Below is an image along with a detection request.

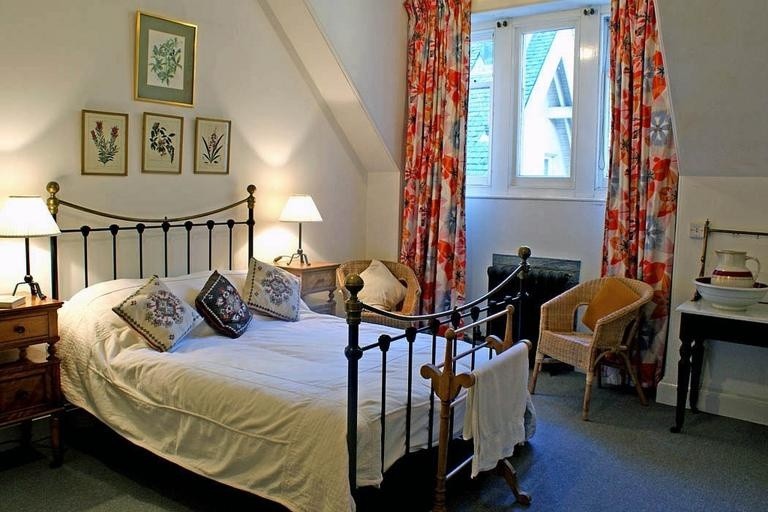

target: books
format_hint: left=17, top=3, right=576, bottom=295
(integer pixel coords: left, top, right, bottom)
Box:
left=1, top=293, right=26, bottom=308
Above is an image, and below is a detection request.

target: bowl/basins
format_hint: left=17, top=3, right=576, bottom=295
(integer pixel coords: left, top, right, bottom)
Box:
left=693, top=276, right=768, bottom=312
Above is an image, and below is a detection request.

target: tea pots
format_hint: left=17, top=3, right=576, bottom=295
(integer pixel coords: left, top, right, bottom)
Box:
left=709, top=248, right=762, bottom=287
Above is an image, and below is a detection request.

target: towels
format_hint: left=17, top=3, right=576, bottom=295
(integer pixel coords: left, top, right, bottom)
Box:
left=468, top=336, right=532, bottom=479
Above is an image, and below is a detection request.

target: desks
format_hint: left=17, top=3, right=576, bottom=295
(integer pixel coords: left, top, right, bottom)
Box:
left=670, top=294, right=768, bottom=434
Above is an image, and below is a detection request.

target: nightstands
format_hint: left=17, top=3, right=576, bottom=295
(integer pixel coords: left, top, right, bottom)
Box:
left=273, top=255, right=339, bottom=319
left=0, top=290, right=66, bottom=450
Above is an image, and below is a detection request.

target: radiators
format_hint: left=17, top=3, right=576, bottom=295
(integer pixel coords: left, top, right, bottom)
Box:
left=485, top=262, right=579, bottom=372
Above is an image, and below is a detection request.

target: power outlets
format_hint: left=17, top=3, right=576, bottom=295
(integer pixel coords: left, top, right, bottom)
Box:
left=687, top=221, right=705, bottom=241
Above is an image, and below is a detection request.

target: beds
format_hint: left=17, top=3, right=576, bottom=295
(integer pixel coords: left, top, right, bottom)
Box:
left=47, top=181, right=540, bottom=509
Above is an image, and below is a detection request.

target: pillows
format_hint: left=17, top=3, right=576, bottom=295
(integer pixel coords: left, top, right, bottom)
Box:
left=351, top=256, right=404, bottom=311
left=111, top=256, right=301, bottom=348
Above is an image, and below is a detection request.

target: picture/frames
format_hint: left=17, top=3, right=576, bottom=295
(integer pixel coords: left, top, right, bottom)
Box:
left=136, top=9, right=200, bottom=109
left=80, top=109, right=234, bottom=175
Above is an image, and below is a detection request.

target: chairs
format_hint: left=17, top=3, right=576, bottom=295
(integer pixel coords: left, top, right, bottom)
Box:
left=529, top=276, right=654, bottom=421
left=333, top=259, right=422, bottom=328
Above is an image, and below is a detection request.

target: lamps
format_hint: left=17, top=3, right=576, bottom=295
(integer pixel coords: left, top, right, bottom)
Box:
left=277, top=194, right=325, bottom=262
left=0, top=194, right=61, bottom=298
